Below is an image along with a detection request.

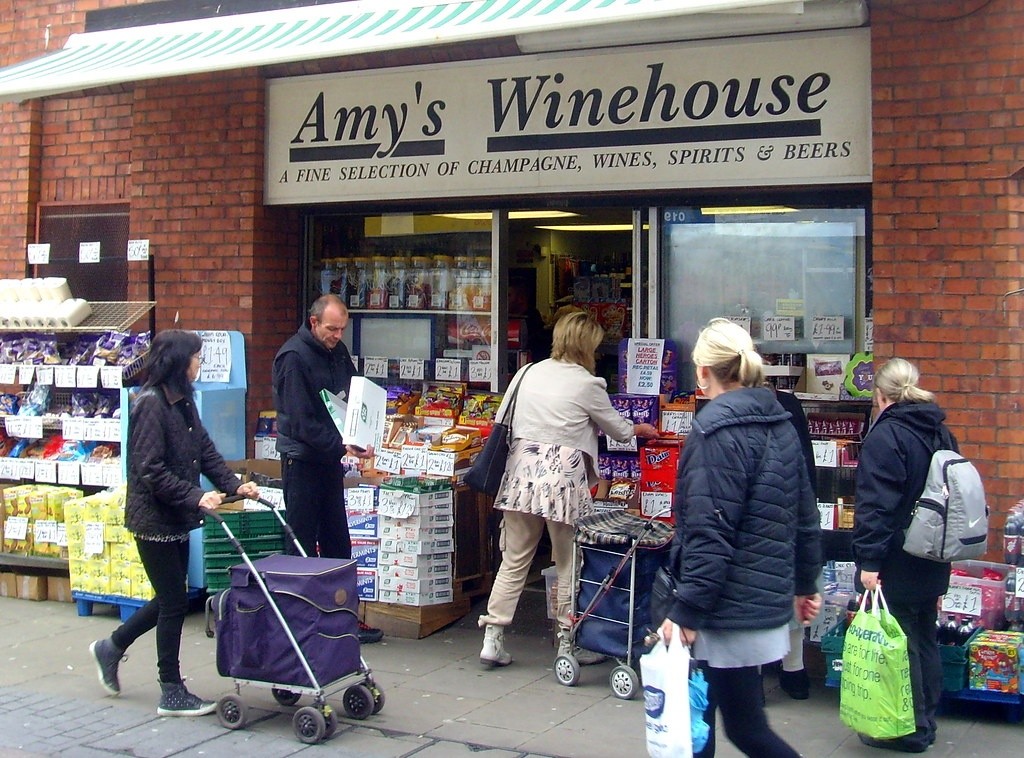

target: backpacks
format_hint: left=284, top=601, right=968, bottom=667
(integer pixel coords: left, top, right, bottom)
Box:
left=881, top=417, right=986, bottom=566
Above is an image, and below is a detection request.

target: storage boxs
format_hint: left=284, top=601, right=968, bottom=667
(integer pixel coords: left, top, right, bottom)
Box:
left=818, top=557, right=1024, bottom=698
left=381, top=389, right=497, bottom=476
left=201, top=486, right=471, bottom=641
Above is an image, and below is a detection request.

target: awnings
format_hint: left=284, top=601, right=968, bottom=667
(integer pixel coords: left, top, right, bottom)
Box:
left=1, top=1, right=802, bottom=104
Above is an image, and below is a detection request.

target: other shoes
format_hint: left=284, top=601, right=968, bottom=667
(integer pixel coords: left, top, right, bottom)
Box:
left=779, top=668, right=810, bottom=700
left=356, top=626, right=383, bottom=643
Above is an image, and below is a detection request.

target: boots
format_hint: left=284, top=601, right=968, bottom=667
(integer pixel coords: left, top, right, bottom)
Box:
left=557, top=623, right=610, bottom=665
left=479, top=623, right=512, bottom=667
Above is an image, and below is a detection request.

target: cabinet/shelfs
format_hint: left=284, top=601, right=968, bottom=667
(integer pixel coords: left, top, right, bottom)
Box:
left=599, top=391, right=874, bottom=520
left=0, top=328, right=151, bottom=571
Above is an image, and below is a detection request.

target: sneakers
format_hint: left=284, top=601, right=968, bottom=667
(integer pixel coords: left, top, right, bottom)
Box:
left=157, top=676, right=217, bottom=716
left=89, top=638, right=128, bottom=696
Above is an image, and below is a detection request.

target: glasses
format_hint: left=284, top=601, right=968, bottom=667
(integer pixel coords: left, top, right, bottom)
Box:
left=194, top=355, right=205, bottom=364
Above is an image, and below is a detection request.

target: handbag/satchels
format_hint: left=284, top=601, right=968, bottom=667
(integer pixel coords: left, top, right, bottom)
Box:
left=639, top=621, right=691, bottom=758
left=464, top=362, right=538, bottom=498
left=839, top=586, right=915, bottom=741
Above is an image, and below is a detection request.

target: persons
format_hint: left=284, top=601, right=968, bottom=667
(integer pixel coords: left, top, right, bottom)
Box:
left=89, top=331, right=262, bottom=717
left=273, top=295, right=384, bottom=643
left=661, top=317, right=825, bottom=758
left=851, top=358, right=959, bottom=753
left=478, top=284, right=662, bottom=667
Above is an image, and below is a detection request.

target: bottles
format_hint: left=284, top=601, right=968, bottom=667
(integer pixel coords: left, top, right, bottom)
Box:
left=846, top=587, right=862, bottom=627
left=935, top=614, right=974, bottom=646
left=317, top=255, right=491, bottom=311
left=1004, top=499, right=1024, bottom=633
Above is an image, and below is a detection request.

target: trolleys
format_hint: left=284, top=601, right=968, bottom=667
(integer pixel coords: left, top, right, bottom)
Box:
left=552, top=506, right=673, bottom=698
left=199, top=496, right=385, bottom=745
